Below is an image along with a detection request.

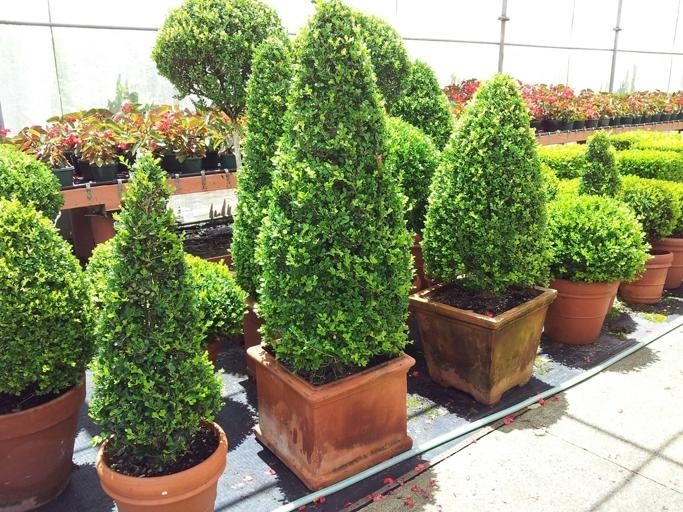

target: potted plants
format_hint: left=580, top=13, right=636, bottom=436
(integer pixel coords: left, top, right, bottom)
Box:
left=542, top=193, right=652, bottom=348
left=614, top=175, right=681, bottom=306
left=85, top=146, right=254, bottom=511
left=1, top=99, right=243, bottom=192
left=246, top=0, right=418, bottom=493
left=225, top=31, right=293, bottom=382
left=440, top=76, right=682, bottom=136
left=405, top=71, right=560, bottom=408
left=82, top=232, right=249, bottom=367
left=1, top=194, right=96, bottom=511
